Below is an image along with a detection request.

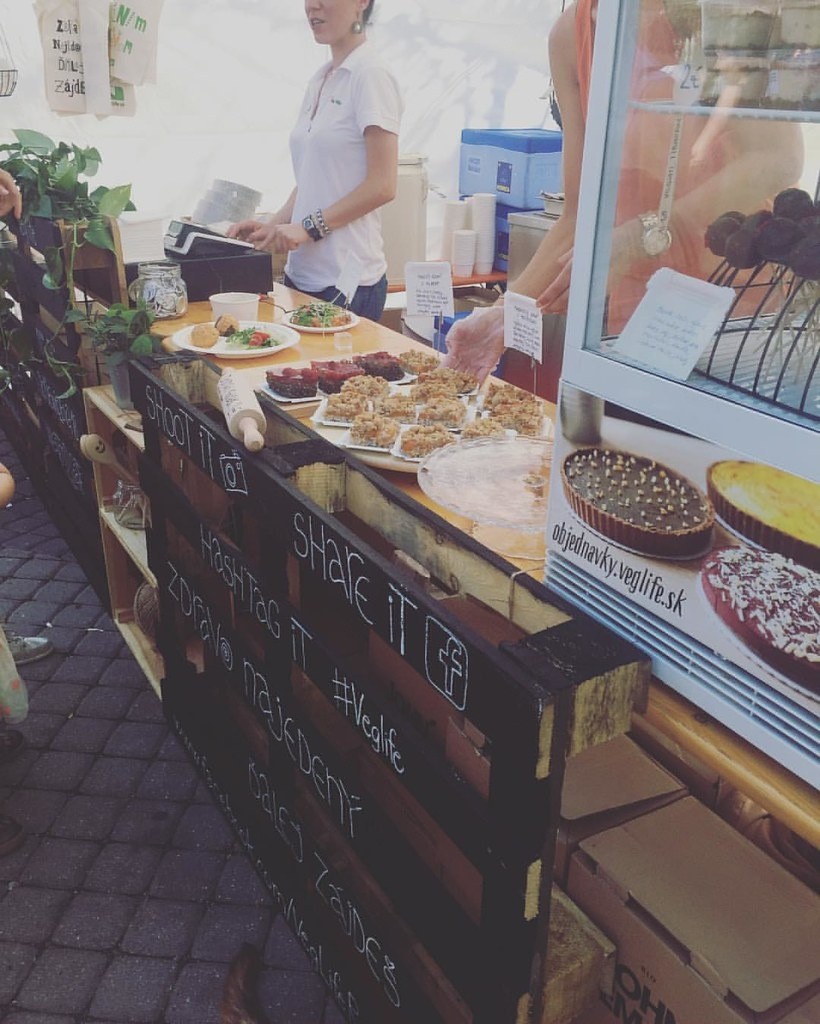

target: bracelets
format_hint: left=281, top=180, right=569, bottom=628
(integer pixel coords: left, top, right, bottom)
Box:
left=316, top=208, right=330, bottom=234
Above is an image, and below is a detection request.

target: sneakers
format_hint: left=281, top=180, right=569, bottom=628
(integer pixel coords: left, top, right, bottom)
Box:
left=6, top=629, right=53, bottom=667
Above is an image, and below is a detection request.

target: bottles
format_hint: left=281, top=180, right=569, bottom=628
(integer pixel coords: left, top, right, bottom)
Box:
left=112, top=480, right=144, bottom=529
left=136, top=261, right=187, bottom=319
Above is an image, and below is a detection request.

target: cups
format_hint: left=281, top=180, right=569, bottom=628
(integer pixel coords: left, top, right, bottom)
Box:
left=559, top=384, right=604, bottom=444
left=441, top=193, right=496, bottom=277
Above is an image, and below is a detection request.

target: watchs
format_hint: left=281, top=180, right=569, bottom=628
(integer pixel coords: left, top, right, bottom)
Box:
left=637, top=210, right=672, bottom=257
left=302, top=215, right=324, bottom=242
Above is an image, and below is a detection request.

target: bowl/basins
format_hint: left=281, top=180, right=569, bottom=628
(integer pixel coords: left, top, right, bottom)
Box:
left=687, top=0, right=820, bottom=113
left=210, top=292, right=261, bottom=321
left=534, top=197, right=565, bottom=216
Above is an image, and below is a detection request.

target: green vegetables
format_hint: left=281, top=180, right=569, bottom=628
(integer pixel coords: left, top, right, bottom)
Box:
left=225, top=302, right=338, bottom=350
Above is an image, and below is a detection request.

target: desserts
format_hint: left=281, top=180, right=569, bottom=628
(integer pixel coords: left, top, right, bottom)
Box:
left=564, top=444, right=820, bottom=699
left=264, top=352, right=543, bottom=458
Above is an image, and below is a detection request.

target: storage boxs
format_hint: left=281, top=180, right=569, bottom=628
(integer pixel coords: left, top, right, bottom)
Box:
left=553, top=711, right=820, bottom=1023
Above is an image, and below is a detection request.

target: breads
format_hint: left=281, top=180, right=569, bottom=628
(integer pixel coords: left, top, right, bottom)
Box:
left=698, top=0, right=820, bottom=112
left=190, top=314, right=239, bottom=348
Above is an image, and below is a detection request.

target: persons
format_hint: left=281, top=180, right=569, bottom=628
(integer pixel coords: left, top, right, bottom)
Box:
left=226, top=0, right=405, bottom=323
left=432, top=0, right=804, bottom=398
left=0, top=167, right=53, bottom=666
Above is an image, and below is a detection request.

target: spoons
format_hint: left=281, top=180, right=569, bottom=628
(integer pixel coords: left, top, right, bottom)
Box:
left=82, top=435, right=136, bottom=483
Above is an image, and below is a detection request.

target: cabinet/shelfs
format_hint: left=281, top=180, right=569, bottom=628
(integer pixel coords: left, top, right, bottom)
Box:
left=84, top=384, right=165, bottom=700
left=547, top=1, right=820, bottom=800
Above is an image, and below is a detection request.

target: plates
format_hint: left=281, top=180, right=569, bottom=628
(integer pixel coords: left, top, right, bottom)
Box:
left=172, top=321, right=300, bottom=356
left=696, top=571, right=820, bottom=702
left=260, top=370, right=549, bottom=464
left=716, top=514, right=769, bottom=553
left=283, top=311, right=359, bottom=333
left=566, top=507, right=716, bottom=561
